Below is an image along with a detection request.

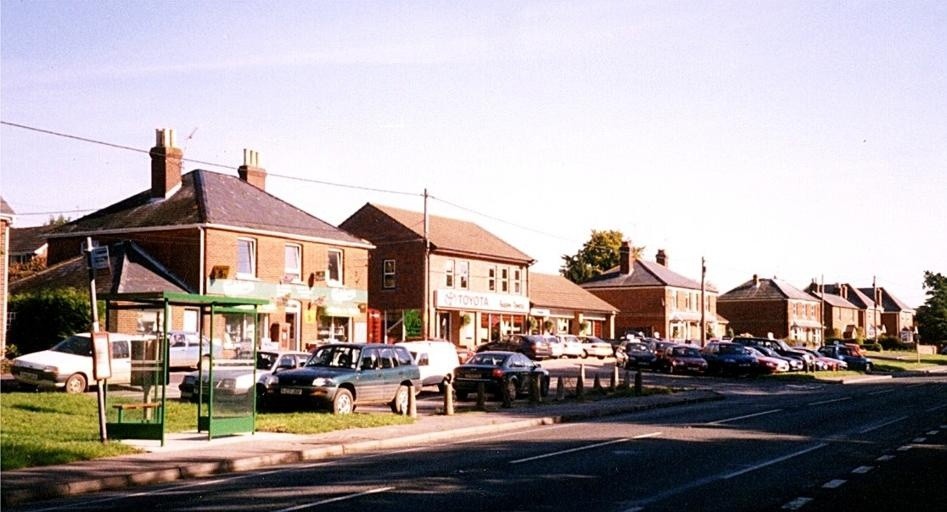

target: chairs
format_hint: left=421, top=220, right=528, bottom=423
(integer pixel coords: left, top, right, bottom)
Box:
left=321, top=351, right=358, bottom=367
left=485, top=359, right=502, bottom=365
